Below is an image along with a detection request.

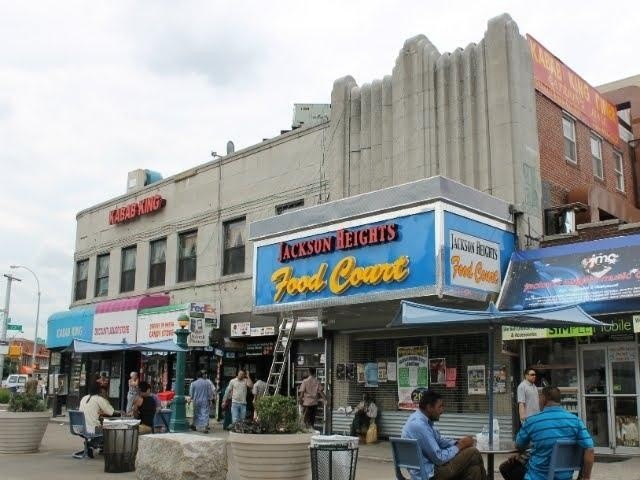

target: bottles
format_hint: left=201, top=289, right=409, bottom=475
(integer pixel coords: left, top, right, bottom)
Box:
left=482, top=424, right=490, bottom=450
left=493, top=441, right=499, bottom=451
left=493, top=418, right=500, bottom=441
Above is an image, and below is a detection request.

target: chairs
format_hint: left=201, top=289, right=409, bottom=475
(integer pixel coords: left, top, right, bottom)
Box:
left=389, top=437, right=428, bottom=480
left=548, top=440, right=585, bottom=480
left=69, top=410, right=104, bottom=459
left=153, top=408, right=173, bottom=434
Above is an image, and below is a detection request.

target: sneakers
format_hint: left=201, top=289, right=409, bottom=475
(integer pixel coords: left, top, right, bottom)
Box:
left=88, top=447, right=95, bottom=458
left=203, top=431, right=208, bottom=433
left=191, top=427, right=195, bottom=431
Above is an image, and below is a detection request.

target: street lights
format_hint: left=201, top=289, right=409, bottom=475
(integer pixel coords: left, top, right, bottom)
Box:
left=9, top=263, right=42, bottom=370
left=169, top=313, right=192, bottom=434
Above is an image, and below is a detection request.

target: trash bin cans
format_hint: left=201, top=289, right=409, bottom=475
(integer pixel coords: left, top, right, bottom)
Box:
left=310, top=435, right=359, bottom=480
left=103, top=419, right=141, bottom=473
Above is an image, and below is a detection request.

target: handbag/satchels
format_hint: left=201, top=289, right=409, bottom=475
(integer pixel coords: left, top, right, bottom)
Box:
left=366, top=419, right=377, bottom=443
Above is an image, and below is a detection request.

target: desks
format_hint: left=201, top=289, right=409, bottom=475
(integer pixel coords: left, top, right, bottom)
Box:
left=100, top=416, right=140, bottom=421
left=469, top=440, right=519, bottom=480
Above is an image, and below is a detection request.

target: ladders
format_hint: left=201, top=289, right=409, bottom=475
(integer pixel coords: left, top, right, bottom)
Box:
left=263, top=318, right=298, bottom=395
left=314, top=368, right=327, bottom=435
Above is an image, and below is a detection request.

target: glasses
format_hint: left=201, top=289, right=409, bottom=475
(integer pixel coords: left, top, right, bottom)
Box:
left=528, top=374, right=537, bottom=376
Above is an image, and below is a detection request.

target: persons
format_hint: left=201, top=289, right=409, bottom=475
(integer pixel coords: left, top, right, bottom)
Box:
left=191, top=304, right=216, bottom=335
left=187, top=369, right=266, bottom=434
left=615, top=416, right=640, bottom=447
left=299, top=367, right=328, bottom=429
left=24, top=373, right=38, bottom=397
left=350, top=391, right=378, bottom=445
left=499, top=385, right=595, bottom=480
left=400, top=391, right=488, bottom=480
left=516, top=368, right=540, bottom=425
left=79, top=372, right=162, bottom=459
